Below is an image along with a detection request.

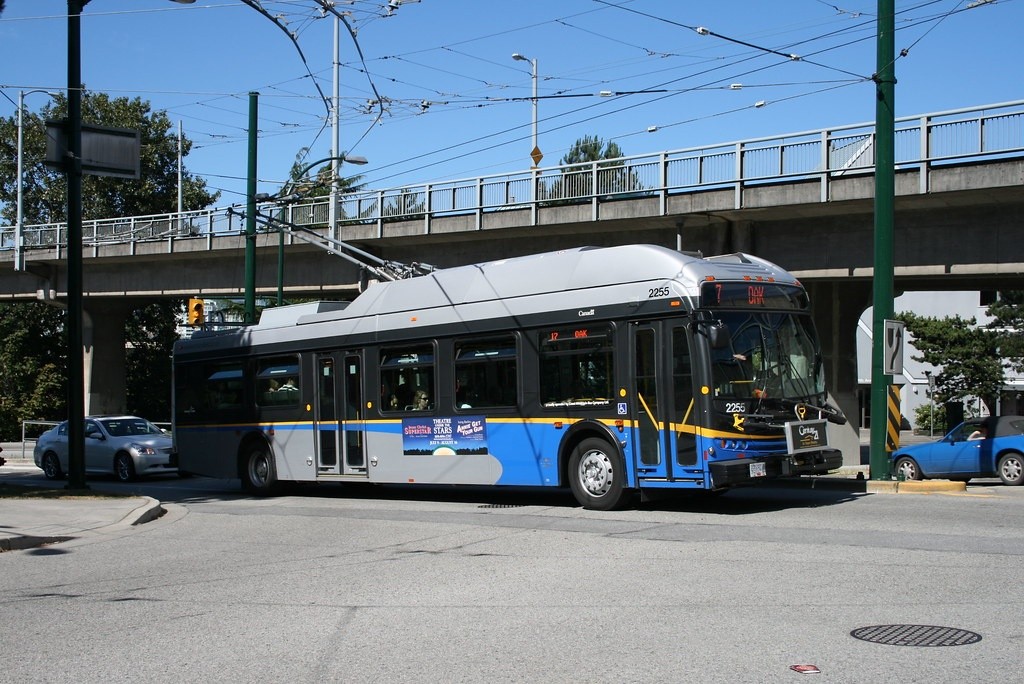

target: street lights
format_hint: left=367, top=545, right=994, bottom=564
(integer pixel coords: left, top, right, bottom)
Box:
left=276, top=156, right=370, bottom=307
left=13, top=88, right=61, bottom=271
left=511, top=51, right=539, bottom=226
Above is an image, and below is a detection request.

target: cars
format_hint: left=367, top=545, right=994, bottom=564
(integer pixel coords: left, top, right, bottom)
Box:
left=33, top=413, right=195, bottom=484
left=887, top=415, right=1024, bottom=487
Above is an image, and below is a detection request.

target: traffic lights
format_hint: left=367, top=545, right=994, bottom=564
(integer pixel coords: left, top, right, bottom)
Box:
left=189, top=299, right=205, bottom=325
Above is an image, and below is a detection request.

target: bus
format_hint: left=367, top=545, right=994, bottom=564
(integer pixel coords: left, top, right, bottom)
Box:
left=170, top=203, right=843, bottom=511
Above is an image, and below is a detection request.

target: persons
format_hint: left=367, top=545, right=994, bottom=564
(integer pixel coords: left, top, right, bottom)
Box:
left=270, top=378, right=298, bottom=392
left=388, top=394, right=399, bottom=411
left=412, top=390, right=430, bottom=411
left=967, top=423, right=992, bottom=442
